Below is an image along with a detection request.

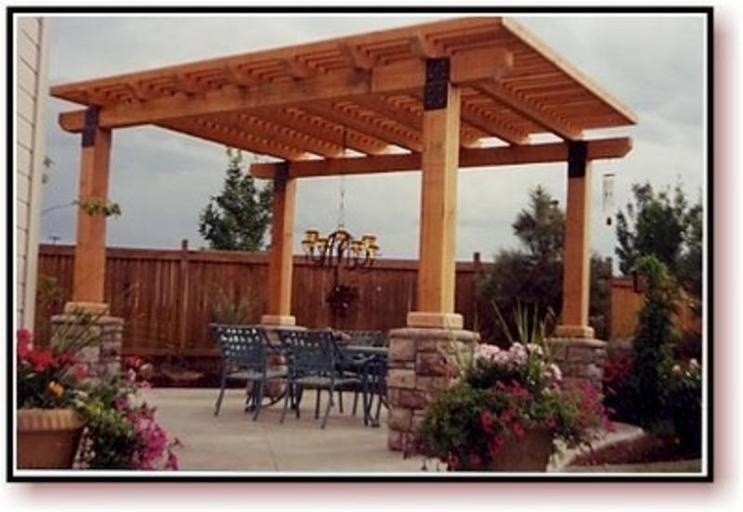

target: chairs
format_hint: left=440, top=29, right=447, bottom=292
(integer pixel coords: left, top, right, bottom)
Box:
left=213, top=323, right=391, bottom=428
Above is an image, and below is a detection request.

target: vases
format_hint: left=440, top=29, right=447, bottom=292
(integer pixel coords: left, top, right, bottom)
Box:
left=18, top=406, right=88, bottom=469
left=487, top=429, right=556, bottom=472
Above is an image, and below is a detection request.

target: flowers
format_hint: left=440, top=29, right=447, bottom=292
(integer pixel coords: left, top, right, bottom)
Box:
left=16, top=329, right=183, bottom=470
left=417, top=353, right=616, bottom=472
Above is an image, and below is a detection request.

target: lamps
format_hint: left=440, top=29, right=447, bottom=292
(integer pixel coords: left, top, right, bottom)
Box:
left=301, top=122, right=378, bottom=274
